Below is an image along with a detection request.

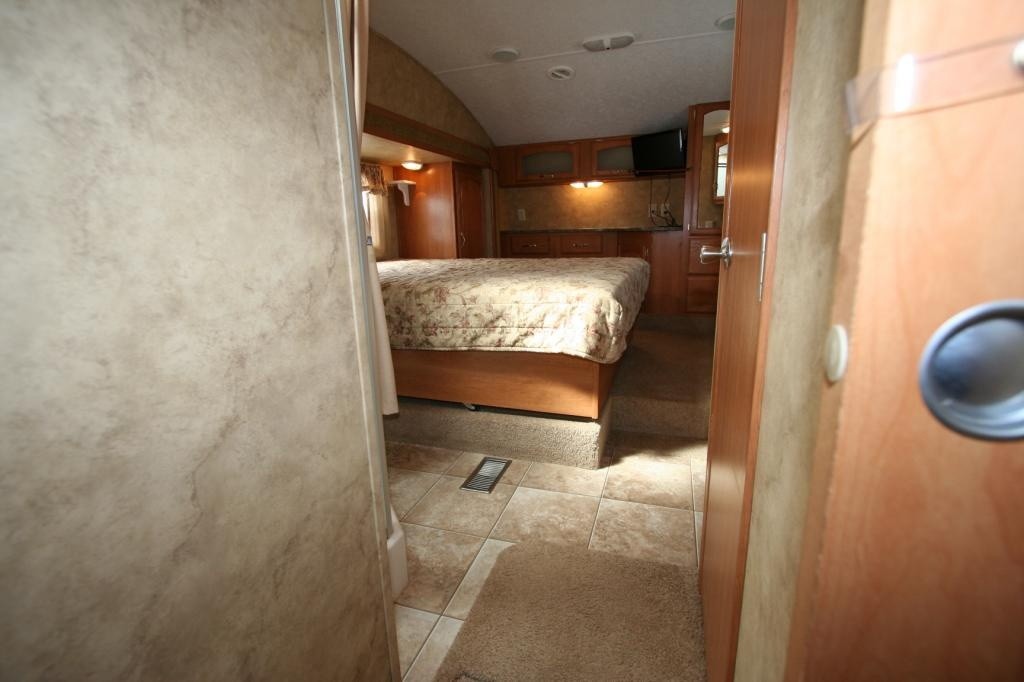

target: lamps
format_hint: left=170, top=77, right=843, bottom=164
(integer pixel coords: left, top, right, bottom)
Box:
left=400, top=160, right=422, bottom=171
left=571, top=181, right=603, bottom=189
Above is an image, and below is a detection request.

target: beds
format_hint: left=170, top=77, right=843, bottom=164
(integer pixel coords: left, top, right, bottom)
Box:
left=371, top=259, right=650, bottom=422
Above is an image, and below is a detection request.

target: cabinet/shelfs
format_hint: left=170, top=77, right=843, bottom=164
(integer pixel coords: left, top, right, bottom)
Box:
left=686, top=237, right=721, bottom=311
left=502, top=235, right=550, bottom=258
left=550, top=234, right=606, bottom=258
left=499, top=136, right=638, bottom=188
left=614, top=230, right=684, bottom=302
left=457, top=165, right=485, bottom=256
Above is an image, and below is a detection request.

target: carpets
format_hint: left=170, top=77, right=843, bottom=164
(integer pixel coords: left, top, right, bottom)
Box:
left=436, top=540, right=707, bottom=682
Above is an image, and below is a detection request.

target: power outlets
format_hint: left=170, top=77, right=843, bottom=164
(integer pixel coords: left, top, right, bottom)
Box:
left=661, top=203, right=671, bottom=217
left=518, top=209, right=526, bottom=222
left=648, top=203, right=657, bottom=218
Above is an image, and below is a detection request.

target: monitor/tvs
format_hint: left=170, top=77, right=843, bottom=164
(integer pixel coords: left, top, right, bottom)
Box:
left=631, top=128, right=686, bottom=175
